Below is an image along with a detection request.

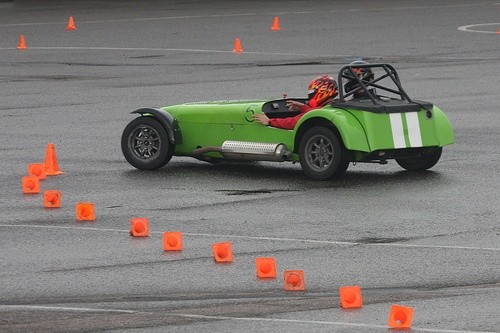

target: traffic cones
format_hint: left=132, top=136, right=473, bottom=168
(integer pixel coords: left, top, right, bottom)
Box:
left=163, top=232, right=183, bottom=251
left=43, top=189, right=61, bottom=208
left=255, top=257, right=277, bottom=278
left=282, top=269, right=306, bottom=291
left=63, top=16, right=79, bottom=30
left=22, top=175, right=41, bottom=194
left=338, top=285, right=364, bottom=309
left=270, top=16, right=282, bottom=31
left=42, top=142, right=63, bottom=176
left=387, top=304, right=416, bottom=330
left=28, top=163, right=46, bottom=180
left=130, top=218, right=149, bottom=238
left=17, top=35, right=28, bottom=49
left=75, top=202, right=96, bottom=221
left=232, top=37, right=244, bottom=53
left=212, top=242, right=235, bottom=263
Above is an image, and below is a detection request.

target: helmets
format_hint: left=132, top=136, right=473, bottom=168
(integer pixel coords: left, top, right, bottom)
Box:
left=308, top=75, right=338, bottom=107
left=343, top=61, right=375, bottom=93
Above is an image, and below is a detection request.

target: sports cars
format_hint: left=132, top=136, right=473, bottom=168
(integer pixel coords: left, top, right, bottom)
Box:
left=120, top=62, right=455, bottom=182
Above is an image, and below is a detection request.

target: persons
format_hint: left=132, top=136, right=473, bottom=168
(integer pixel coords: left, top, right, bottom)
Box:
left=251, top=74, right=338, bottom=130
left=341, top=60, right=382, bottom=101
